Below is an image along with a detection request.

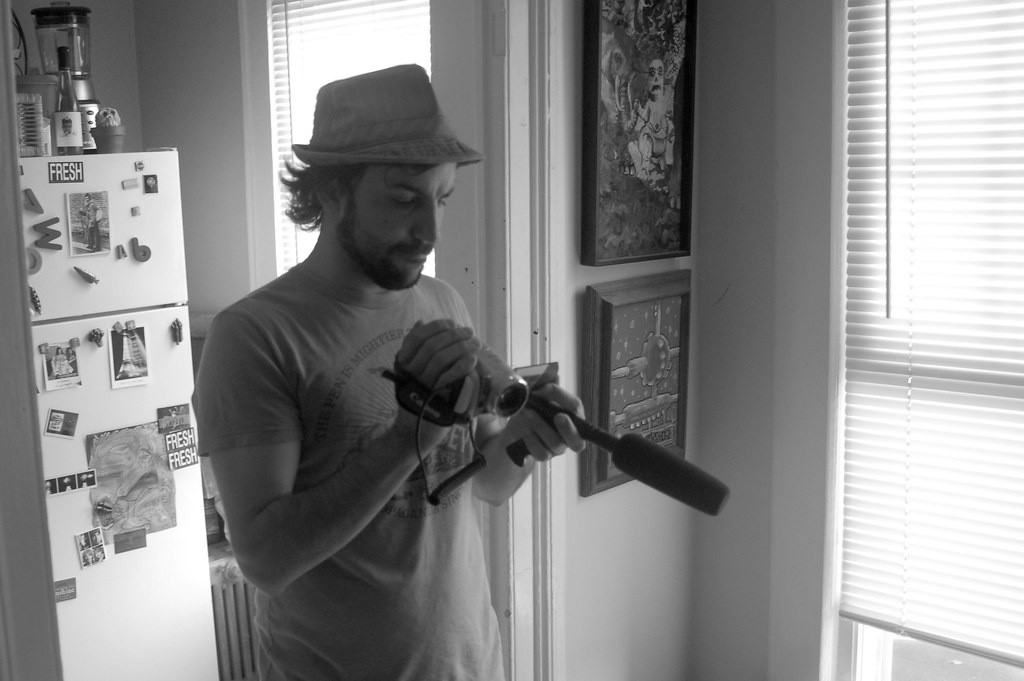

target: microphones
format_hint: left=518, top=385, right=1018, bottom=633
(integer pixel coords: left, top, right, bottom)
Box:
left=536, top=403, right=731, bottom=515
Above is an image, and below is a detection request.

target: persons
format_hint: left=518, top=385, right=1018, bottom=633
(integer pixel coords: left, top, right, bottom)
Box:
left=193, top=66, right=585, bottom=681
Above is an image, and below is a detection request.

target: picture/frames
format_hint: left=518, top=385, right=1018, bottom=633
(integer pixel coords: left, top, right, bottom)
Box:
left=581, top=0, right=697, bottom=267
left=580, top=269, right=692, bottom=498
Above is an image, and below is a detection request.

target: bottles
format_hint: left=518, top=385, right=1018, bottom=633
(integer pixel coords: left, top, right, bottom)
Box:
left=53, top=47, right=84, bottom=154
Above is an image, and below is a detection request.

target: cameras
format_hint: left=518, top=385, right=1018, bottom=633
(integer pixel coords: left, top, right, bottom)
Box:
left=449, top=339, right=559, bottom=418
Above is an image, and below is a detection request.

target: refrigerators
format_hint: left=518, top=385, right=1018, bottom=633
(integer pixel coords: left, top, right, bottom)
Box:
left=18, top=147, right=218, bottom=680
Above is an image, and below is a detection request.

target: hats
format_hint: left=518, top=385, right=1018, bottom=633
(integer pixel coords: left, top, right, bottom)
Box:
left=290, top=65, right=487, bottom=166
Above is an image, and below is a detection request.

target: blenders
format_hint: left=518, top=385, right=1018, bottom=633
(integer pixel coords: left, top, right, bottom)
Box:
left=30, top=6, right=101, bottom=153
